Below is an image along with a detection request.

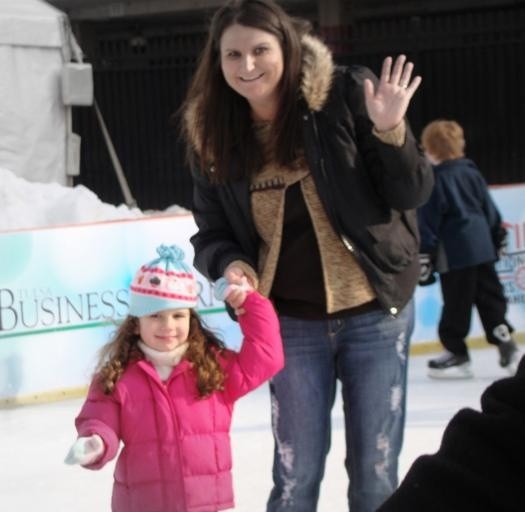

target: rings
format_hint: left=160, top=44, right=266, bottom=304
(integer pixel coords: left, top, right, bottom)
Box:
left=398, top=83, right=408, bottom=89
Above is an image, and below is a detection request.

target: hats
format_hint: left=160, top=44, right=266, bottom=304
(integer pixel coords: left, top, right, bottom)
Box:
left=127, top=245, right=198, bottom=318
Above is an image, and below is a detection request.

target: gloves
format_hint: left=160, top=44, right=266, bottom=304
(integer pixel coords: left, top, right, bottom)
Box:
left=414, top=253, right=436, bottom=286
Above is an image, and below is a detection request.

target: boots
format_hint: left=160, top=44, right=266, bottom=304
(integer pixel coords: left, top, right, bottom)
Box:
left=492, top=323, right=520, bottom=367
left=429, top=352, right=469, bottom=368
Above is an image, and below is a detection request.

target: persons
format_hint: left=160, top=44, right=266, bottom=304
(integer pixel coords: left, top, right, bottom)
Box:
left=181, top=1, right=434, bottom=509
left=63, top=238, right=289, bottom=511
left=372, top=349, right=525, bottom=512
left=413, top=119, right=520, bottom=370
